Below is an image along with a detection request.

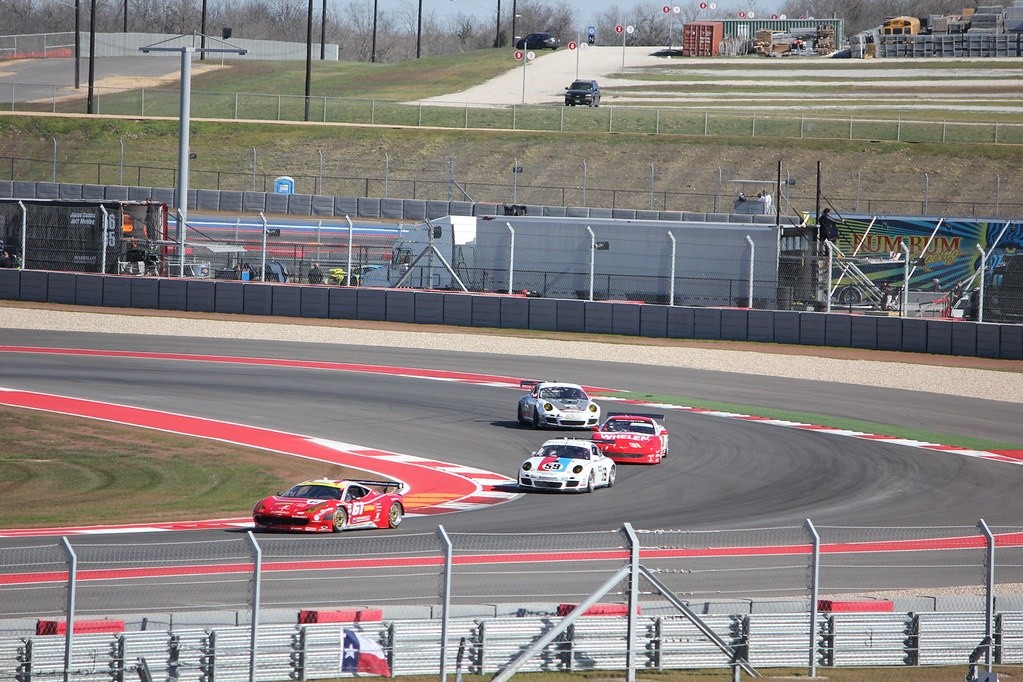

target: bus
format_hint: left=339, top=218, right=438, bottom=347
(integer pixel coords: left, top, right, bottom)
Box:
left=882, top=16, right=921, bottom=44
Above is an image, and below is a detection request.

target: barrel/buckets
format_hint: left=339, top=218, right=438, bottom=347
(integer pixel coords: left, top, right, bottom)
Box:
left=777, top=287, right=793, bottom=310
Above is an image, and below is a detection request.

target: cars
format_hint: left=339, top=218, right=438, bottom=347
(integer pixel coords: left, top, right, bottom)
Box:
left=344, top=265, right=385, bottom=287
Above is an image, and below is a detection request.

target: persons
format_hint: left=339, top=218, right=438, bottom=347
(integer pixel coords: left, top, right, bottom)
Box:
left=308, top=263, right=323, bottom=284
left=736, top=190, right=772, bottom=214
left=814, top=208, right=847, bottom=257
left=3, top=250, right=22, bottom=268
left=574, top=388, right=582, bottom=399
left=233, top=262, right=241, bottom=279
left=577, top=450, right=587, bottom=456
left=952, top=280, right=964, bottom=305
left=881, top=279, right=893, bottom=309
left=932, top=277, right=941, bottom=293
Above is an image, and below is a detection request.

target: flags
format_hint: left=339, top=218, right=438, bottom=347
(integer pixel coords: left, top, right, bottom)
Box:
left=339, top=629, right=392, bottom=678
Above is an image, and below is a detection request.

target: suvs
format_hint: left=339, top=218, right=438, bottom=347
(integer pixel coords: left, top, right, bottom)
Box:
left=565, top=79, right=601, bottom=107
left=516, top=31, right=561, bottom=49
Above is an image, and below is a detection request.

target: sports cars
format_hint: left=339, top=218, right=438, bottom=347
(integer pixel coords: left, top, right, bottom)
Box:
left=591, top=412, right=668, bottom=464
left=518, top=437, right=616, bottom=493
left=253, top=477, right=404, bottom=533
left=518, top=380, right=601, bottom=430
